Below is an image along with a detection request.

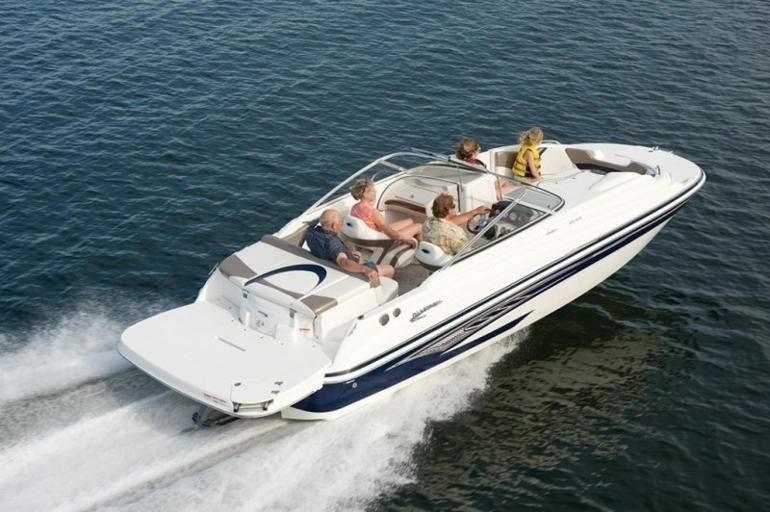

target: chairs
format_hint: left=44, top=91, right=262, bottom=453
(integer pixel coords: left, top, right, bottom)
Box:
left=414, top=241, right=452, bottom=275
left=341, top=215, right=418, bottom=269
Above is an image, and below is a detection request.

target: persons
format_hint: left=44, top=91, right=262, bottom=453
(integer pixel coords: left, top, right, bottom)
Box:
left=347, top=175, right=424, bottom=252
left=304, top=208, right=395, bottom=287
left=510, top=126, right=545, bottom=187
left=458, top=134, right=516, bottom=197
left=421, top=191, right=491, bottom=256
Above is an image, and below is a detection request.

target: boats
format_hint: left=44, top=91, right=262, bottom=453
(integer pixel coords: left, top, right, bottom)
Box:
left=118, top=140, right=706, bottom=428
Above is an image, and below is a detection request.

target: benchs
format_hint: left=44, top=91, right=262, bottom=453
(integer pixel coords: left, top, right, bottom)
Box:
left=261, top=234, right=400, bottom=306
left=493, top=176, right=527, bottom=200
